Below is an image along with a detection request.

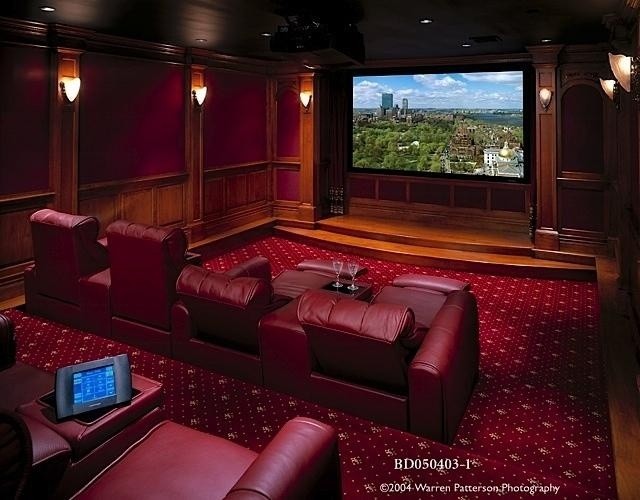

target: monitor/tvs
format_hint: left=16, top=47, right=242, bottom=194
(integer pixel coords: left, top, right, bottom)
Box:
left=345, top=62, right=536, bottom=183
left=55, top=353, right=131, bottom=420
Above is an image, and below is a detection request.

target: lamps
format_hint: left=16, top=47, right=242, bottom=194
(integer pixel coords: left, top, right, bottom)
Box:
left=598, top=50, right=640, bottom=109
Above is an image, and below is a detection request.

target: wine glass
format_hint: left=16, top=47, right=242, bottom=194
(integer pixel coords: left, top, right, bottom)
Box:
left=346, top=264, right=359, bottom=290
left=332, top=262, right=344, bottom=288
